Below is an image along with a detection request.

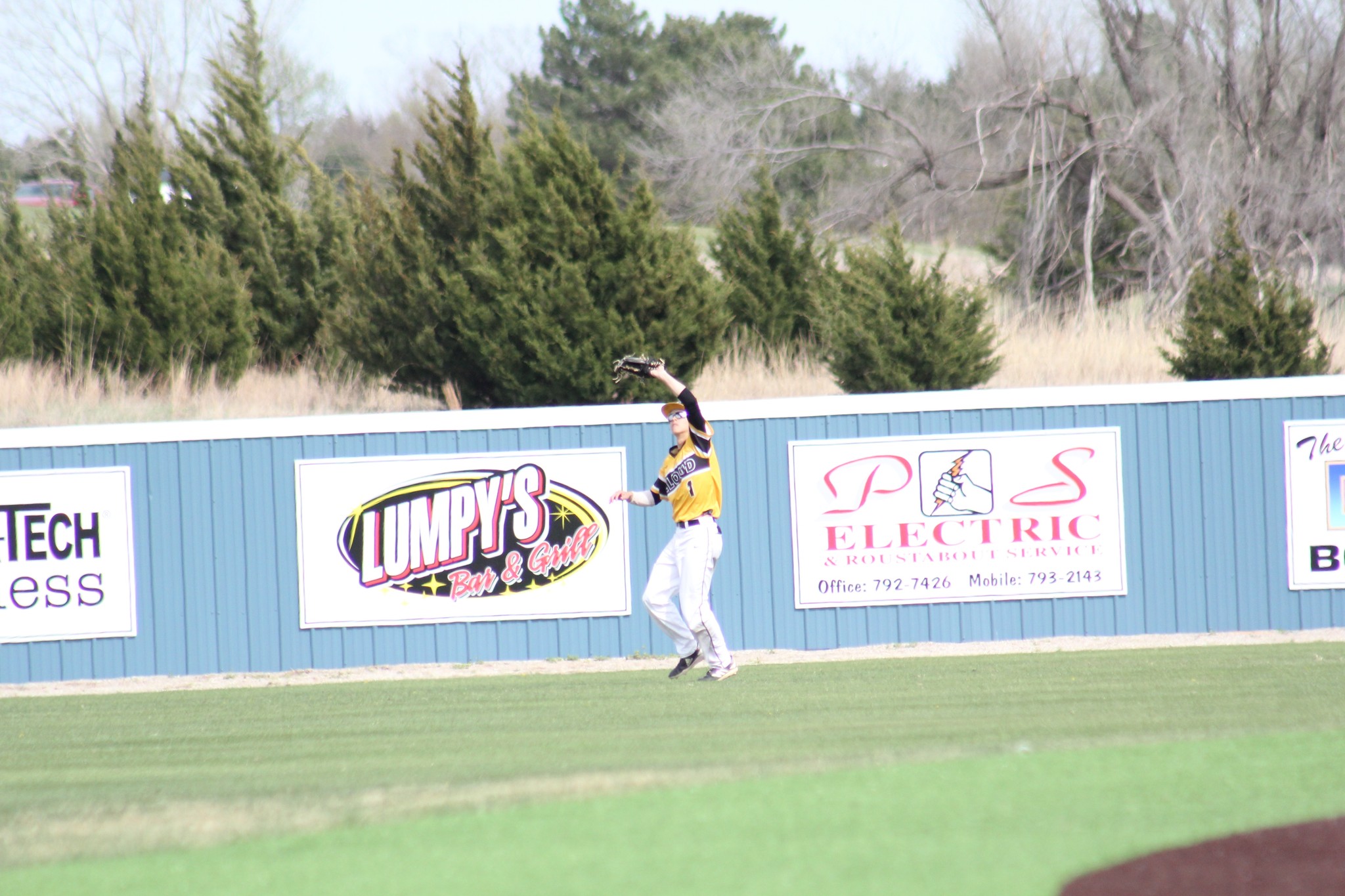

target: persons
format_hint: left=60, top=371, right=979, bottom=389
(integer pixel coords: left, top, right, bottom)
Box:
left=608, top=363, right=739, bottom=682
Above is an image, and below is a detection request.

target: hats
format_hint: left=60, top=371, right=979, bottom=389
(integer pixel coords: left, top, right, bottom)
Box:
left=661, top=403, right=685, bottom=419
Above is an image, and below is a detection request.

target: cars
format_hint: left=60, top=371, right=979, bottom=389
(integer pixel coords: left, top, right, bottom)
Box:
left=0, top=176, right=113, bottom=210
left=126, top=167, right=192, bottom=207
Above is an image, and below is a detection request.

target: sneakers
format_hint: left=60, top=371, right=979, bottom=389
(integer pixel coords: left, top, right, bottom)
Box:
left=668, top=643, right=705, bottom=679
left=697, top=655, right=738, bottom=681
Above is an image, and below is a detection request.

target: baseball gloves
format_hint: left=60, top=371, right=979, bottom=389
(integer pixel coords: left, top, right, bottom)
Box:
left=614, top=355, right=653, bottom=379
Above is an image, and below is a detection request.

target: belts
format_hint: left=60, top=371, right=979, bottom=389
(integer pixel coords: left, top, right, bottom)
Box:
left=676, top=517, right=716, bottom=528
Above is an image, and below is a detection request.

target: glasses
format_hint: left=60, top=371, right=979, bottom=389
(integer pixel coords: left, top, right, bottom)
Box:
left=668, top=411, right=687, bottom=423
